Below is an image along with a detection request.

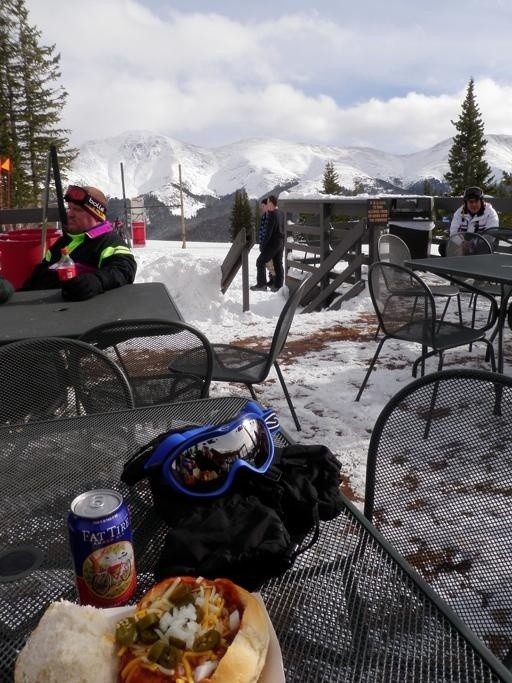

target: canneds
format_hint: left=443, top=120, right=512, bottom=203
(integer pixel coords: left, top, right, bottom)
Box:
left=67, top=489, right=137, bottom=608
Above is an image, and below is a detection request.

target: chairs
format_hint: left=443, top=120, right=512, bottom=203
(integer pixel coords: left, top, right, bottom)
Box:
left=375, top=234, right=463, bottom=340
left=441, top=232, right=505, bottom=352
left=168, top=272, right=311, bottom=431
left=354, top=262, right=499, bottom=402
left=69, top=319, right=213, bottom=415
left=219, top=226, right=512, bottom=378
left=2, top=338, right=134, bottom=425
left=364, top=368, right=511, bottom=670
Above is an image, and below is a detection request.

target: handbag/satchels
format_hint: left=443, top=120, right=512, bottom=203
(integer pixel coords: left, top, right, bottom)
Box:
left=129, top=443, right=343, bottom=590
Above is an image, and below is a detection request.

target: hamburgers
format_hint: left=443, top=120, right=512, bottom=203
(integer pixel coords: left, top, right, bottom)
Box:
left=115, top=576, right=270, bottom=683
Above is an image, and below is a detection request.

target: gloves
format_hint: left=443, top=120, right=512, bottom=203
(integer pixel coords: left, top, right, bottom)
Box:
left=64, top=274, right=103, bottom=302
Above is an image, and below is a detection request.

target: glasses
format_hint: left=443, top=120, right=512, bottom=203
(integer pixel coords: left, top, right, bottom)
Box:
left=69, top=187, right=106, bottom=222
left=122, top=407, right=278, bottom=497
left=464, top=190, right=482, bottom=197
left=65, top=185, right=91, bottom=206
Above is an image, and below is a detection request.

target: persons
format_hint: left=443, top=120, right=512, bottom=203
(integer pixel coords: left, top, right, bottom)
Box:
left=114, top=216, right=123, bottom=231
left=438, top=186, right=499, bottom=292
left=258, top=198, right=277, bottom=287
left=10, top=186, right=137, bottom=302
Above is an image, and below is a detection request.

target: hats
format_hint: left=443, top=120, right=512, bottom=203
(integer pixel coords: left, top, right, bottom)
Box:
left=463, top=187, right=483, bottom=201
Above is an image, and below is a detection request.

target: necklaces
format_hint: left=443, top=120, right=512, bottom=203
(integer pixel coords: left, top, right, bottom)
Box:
left=250, top=195, right=286, bottom=291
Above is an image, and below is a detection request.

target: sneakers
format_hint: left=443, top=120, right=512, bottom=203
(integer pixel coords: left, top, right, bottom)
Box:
left=271, top=283, right=281, bottom=291
left=250, top=284, right=267, bottom=292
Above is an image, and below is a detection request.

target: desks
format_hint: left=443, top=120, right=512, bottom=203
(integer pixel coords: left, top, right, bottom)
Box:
left=0, top=396, right=510, bottom=682
left=0, top=282, right=186, bottom=348
left=404, top=252, right=512, bottom=374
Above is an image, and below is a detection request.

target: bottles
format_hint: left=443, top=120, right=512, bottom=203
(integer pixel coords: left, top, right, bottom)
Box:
left=56, top=245, right=79, bottom=300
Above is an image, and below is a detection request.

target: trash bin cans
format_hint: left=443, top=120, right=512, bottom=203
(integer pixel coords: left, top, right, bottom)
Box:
left=132, top=221, right=145, bottom=245
left=0, top=228, right=64, bottom=292
left=389, top=211, right=435, bottom=273
